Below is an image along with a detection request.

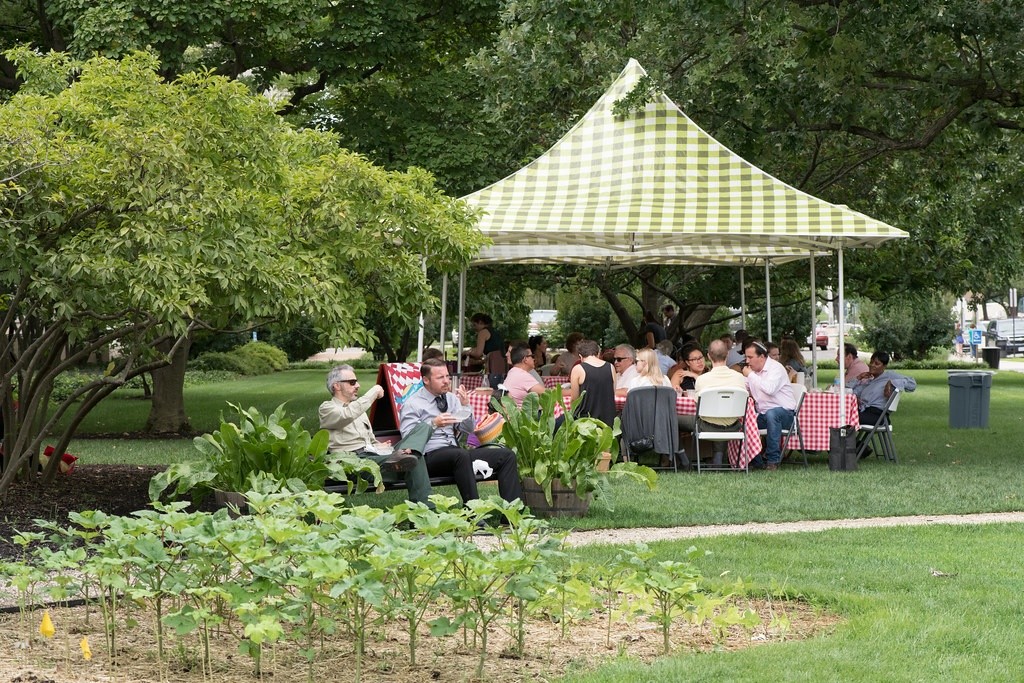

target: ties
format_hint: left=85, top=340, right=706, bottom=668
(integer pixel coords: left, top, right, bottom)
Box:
left=434, top=394, right=448, bottom=412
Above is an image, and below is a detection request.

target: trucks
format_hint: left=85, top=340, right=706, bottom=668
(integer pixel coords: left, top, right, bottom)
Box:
left=953, top=318, right=1023, bottom=353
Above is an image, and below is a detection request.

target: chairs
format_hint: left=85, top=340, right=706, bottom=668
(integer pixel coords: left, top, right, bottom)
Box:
left=681, top=387, right=749, bottom=476
left=621, top=386, right=679, bottom=474
left=758, top=383, right=808, bottom=469
left=855, top=387, right=900, bottom=463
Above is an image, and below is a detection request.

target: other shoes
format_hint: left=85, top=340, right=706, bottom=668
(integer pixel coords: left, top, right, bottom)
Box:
left=383, top=450, right=418, bottom=473
left=675, top=449, right=690, bottom=470
left=712, top=452, right=723, bottom=472
left=498, top=514, right=511, bottom=528
left=856, top=440, right=873, bottom=460
left=764, top=462, right=778, bottom=471
left=467, top=516, right=486, bottom=531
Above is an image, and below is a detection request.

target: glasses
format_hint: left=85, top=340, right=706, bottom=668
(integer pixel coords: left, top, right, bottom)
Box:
left=634, top=360, right=646, bottom=365
left=526, top=354, right=535, bottom=358
left=337, top=379, right=357, bottom=385
left=613, top=357, right=627, bottom=362
left=687, top=356, right=704, bottom=363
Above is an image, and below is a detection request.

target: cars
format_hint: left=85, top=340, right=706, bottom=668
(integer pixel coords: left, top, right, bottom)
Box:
left=805, top=322, right=860, bottom=350
left=527, top=309, right=561, bottom=332
left=451, top=315, right=468, bottom=344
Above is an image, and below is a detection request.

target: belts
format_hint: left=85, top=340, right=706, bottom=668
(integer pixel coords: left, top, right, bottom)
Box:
left=699, top=417, right=739, bottom=430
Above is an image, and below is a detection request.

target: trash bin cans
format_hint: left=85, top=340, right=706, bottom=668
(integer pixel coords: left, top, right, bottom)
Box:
left=981, top=347, right=1001, bottom=369
left=948, top=371, right=992, bottom=429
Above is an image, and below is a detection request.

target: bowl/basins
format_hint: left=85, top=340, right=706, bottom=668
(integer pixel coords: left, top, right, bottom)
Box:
left=686, top=389, right=695, bottom=398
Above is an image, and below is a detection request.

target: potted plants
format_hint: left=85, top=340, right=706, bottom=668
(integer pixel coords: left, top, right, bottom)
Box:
left=148, top=398, right=386, bottom=521
left=489, top=382, right=659, bottom=520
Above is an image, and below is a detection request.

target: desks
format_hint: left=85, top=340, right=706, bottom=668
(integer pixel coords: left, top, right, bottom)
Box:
left=460, top=374, right=860, bottom=471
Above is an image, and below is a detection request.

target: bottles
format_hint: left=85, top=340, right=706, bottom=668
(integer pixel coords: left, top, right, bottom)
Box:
left=834, top=374, right=840, bottom=394
left=481, top=374, right=490, bottom=388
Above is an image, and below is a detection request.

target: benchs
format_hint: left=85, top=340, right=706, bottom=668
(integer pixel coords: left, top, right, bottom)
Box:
left=307, top=430, right=498, bottom=493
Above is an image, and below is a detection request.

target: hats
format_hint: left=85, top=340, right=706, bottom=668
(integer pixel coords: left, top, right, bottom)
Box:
left=752, top=340, right=768, bottom=353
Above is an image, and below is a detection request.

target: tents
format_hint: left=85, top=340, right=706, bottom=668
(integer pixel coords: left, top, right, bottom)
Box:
left=418, top=58, right=909, bottom=471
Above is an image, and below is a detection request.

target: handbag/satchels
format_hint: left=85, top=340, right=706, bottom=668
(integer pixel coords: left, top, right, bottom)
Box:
left=829, top=424, right=857, bottom=472
left=629, top=437, right=655, bottom=454
left=956, top=335, right=964, bottom=343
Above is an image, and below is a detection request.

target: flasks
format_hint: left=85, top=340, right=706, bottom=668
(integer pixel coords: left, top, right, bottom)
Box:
left=451, top=376, right=459, bottom=395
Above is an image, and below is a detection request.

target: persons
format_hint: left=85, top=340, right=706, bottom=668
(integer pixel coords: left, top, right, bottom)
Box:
left=503, top=340, right=565, bottom=426
left=400, top=358, right=525, bottom=530
left=826, top=343, right=874, bottom=390
left=550, top=333, right=585, bottom=375
left=969, top=324, right=977, bottom=357
left=570, top=341, right=617, bottom=430
left=742, top=340, right=797, bottom=470
left=619, top=349, right=671, bottom=467
left=528, top=336, right=547, bottom=368
left=422, top=348, right=443, bottom=362
left=463, top=314, right=506, bottom=388
left=603, top=305, right=710, bottom=397
left=779, top=339, right=807, bottom=376
left=954, top=323, right=964, bottom=353
left=765, top=342, right=797, bottom=383
left=677, top=339, right=746, bottom=470
left=846, top=351, right=917, bottom=460
left=319, top=364, right=437, bottom=510
left=720, top=329, right=757, bottom=372
